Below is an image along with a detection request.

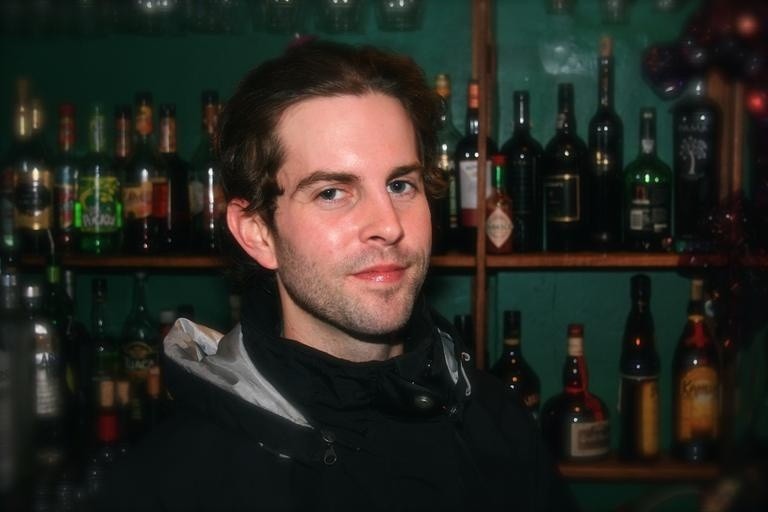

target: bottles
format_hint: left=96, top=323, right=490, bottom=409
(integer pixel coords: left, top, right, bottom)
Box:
left=2, top=58, right=729, bottom=255
left=544, top=324, right=606, bottom=467
left=622, top=291, right=658, bottom=467
left=491, top=310, right=541, bottom=464
left=8, top=267, right=471, bottom=469
left=674, top=277, right=727, bottom=469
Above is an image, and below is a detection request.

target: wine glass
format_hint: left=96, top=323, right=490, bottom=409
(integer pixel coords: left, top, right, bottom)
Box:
left=114, top=0, right=679, bottom=38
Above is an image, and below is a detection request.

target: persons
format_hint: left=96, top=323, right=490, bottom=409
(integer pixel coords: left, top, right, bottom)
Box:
left=99, top=40, right=573, bottom=512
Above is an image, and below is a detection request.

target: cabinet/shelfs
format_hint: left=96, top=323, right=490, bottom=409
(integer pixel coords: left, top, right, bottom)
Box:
left=0, top=0, right=768, bottom=479
